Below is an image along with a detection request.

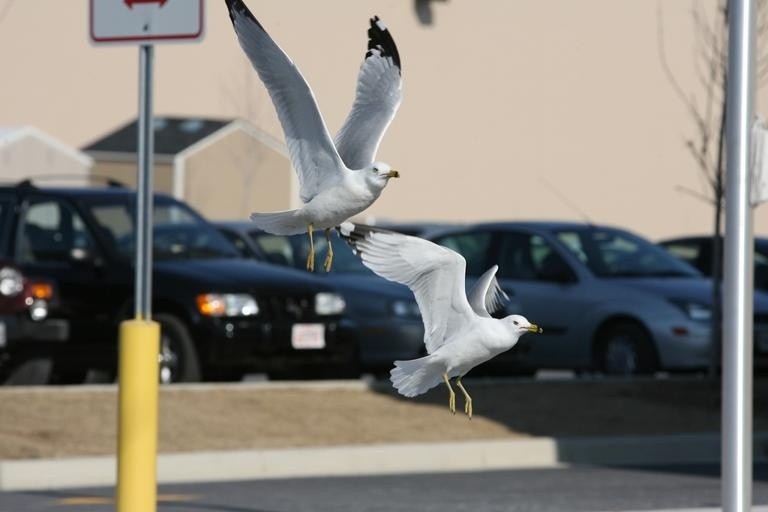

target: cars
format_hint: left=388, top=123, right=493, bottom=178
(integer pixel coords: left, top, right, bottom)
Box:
left=0, top=264, right=72, bottom=385
left=417, top=218, right=768, bottom=380
left=117, top=218, right=539, bottom=380
left=613, top=233, right=768, bottom=293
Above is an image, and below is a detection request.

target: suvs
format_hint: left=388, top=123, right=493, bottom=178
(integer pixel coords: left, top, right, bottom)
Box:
left=1, top=174, right=363, bottom=389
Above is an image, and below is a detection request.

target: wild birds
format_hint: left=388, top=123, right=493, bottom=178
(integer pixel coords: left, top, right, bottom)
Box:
left=225, top=0, right=403, bottom=273
left=333, top=219, right=544, bottom=421
